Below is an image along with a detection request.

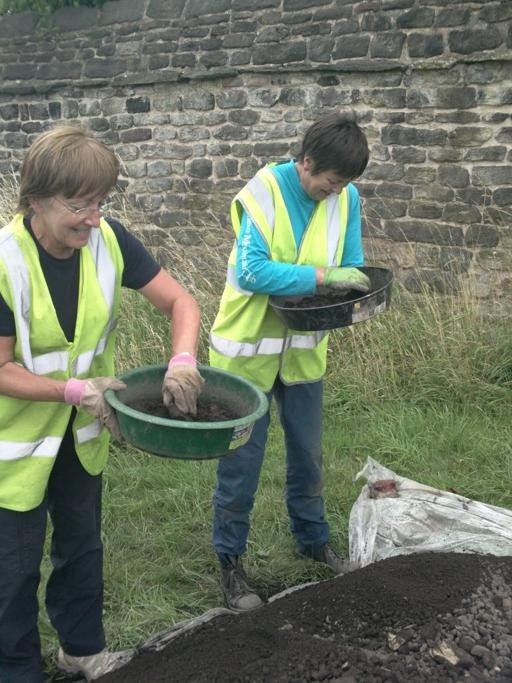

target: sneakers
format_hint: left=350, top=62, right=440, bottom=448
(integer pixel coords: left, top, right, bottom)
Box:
left=217, top=552, right=263, bottom=612
left=57, top=645, right=118, bottom=680
left=294, top=542, right=357, bottom=574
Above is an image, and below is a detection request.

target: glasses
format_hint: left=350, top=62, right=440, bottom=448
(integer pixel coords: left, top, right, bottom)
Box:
left=53, top=196, right=115, bottom=216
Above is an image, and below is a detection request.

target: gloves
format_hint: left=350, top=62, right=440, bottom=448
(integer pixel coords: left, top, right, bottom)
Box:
left=161, top=354, right=204, bottom=416
left=65, top=376, right=128, bottom=445
left=323, top=266, right=370, bottom=292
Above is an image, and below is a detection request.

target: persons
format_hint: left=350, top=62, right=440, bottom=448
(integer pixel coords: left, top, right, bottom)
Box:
left=204, top=111, right=374, bottom=612
left=0, top=126, right=204, bottom=683
